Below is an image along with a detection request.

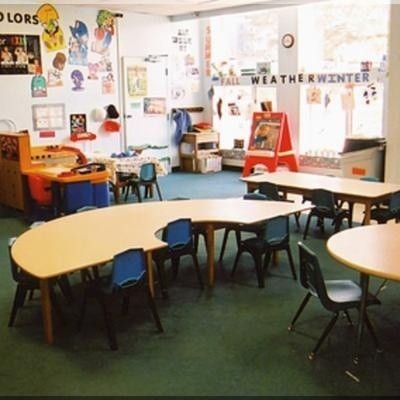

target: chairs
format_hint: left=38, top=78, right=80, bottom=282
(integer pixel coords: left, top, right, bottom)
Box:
left=9, top=238, right=62, bottom=324
left=29, top=221, right=85, bottom=295
left=287, top=239, right=387, bottom=361
left=230, top=216, right=296, bottom=288
left=244, top=170, right=400, bottom=239
left=153, top=219, right=204, bottom=289
left=86, top=247, right=165, bottom=348
left=123, top=163, right=163, bottom=203
left=77, top=206, right=103, bottom=276
left=218, top=194, right=268, bottom=261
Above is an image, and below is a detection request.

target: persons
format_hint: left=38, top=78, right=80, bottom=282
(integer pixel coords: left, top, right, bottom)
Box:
left=71, top=70, right=83, bottom=90
left=255, top=123, right=270, bottom=142
left=52, top=53, right=66, bottom=79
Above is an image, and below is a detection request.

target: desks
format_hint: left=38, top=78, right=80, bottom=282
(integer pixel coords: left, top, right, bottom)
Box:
left=237, top=173, right=400, bottom=230
left=326, top=223, right=399, bottom=370
left=10, top=199, right=317, bottom=341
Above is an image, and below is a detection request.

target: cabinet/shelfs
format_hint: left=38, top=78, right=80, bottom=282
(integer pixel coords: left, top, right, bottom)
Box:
left=178, top=131, right=220, bottom=174
